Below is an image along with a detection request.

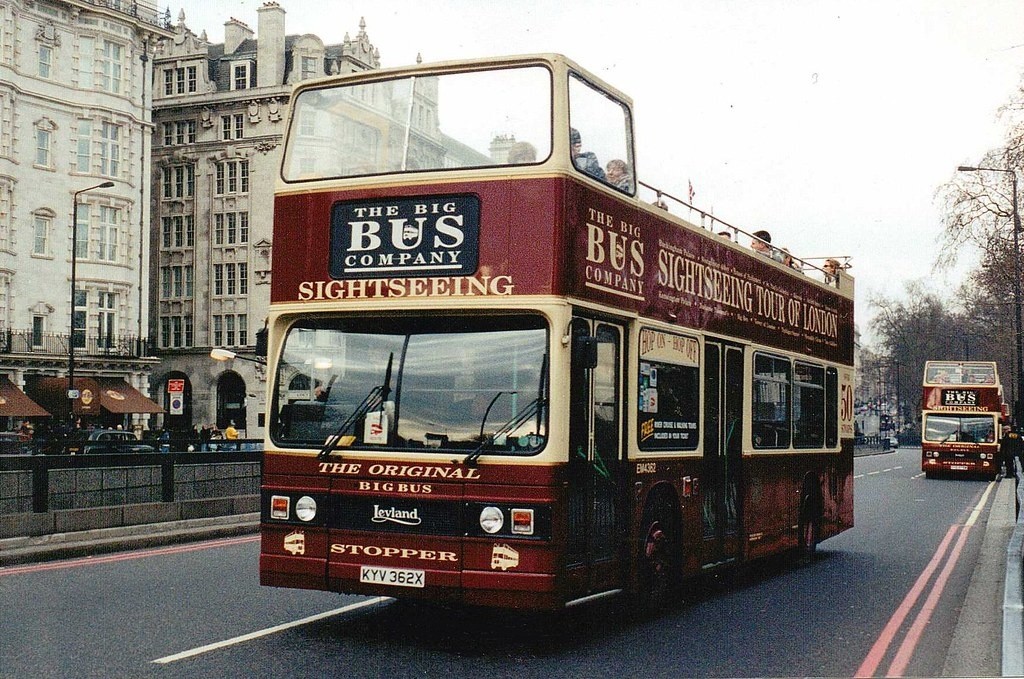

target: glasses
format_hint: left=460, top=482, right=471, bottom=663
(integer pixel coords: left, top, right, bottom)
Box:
left=823, top=265, right=832, bottom=267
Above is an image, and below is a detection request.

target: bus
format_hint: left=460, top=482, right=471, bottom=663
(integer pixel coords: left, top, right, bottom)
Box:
left=920, top=361, right=1012, bottom=475
left=255, top=52, right=856, bottom=613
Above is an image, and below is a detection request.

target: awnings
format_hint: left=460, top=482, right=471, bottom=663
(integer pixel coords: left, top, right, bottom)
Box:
left=0, top=376, right=52, bottom=417
left=96, top=376, right=167, bottom=414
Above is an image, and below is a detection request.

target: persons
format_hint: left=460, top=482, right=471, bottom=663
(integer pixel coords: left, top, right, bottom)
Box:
left=12, top=417, right=129, bottom=454
left=605, top=159, right=629, bottom=194
left=995, top=426, right=1024, bottom=481
left=570, top=127, right=605, bottom=182
left=188, top=422, right=240, bottom=452
left=507, top=142, right=537, bottom=163
left=779, top=249, right=805, bottom=273
left=750, top=231, right=782, bottom=264
left=822, top=259, right=843, bottom=289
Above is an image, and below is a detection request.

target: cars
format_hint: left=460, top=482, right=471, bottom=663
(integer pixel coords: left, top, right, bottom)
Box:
left=0, top=431, right=29, bottom=456
left=885, top=437, right=899, bottom=449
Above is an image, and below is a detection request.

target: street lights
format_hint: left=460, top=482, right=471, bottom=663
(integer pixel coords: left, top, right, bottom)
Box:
left=958, top=166, right=1024, bottom=453
left=68, top=181, right=116, bottom=434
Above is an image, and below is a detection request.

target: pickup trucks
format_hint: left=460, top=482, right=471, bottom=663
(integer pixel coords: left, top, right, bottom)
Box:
left=44, top=428, right=156, bottom=454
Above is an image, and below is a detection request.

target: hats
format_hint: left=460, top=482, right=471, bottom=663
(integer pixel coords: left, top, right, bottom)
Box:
left=228, top=420, right=235, bottom=425
